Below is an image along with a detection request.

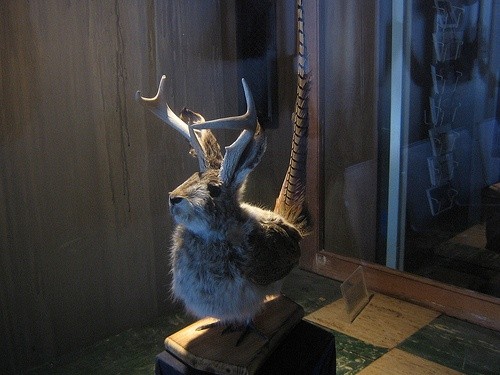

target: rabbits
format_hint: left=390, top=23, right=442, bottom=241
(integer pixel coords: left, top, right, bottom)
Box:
left=166, top=108, right=305, bottom=323
